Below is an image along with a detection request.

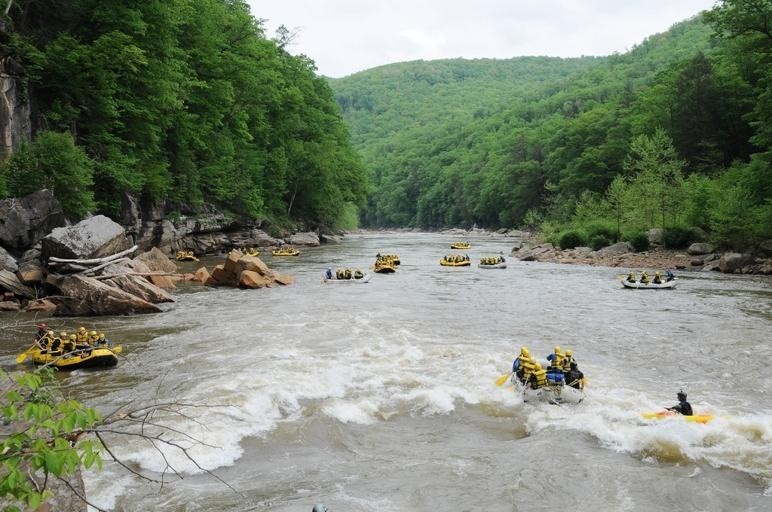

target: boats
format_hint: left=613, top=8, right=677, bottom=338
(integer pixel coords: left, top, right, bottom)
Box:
left=640, top=410, right=732, bottom=424
left=272, top=251, right=299, bottom=256
left=320, top=253, right=401, bottom=284
left=225, top=252, right=260, bottom=257
left=451, top=245, right=471, bottom=249
left=621, top=278, right=679, bottom=289
left=32, top=347, right=118, bottom=371
left=439, top=260, right=507, bottom=269
left=511, top=359, right=584, bottom=405
left=176, top=255, right=196, bottom=261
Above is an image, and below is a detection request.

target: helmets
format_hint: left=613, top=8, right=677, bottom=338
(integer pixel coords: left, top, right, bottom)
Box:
left=529, top=357, right=535, bottom=364
left=78, top=327, right=85, bottom=333
left=99, top=333, right=105, bottom=338
left=523, top=351, right=529, bottom=357
left=520, top=347, right=527, bottom=353
left=565, top=350, right=578, bottom=368
left=534, top=363, right=541, bottom=370
left=554, top=346, right=560, bottom=353
left=37, top=323, right=54, bottom=335
left=90, top=330, right=97, bottom=336
left=69, top=334, right=77, bottom=340
left=59, top=332, right=67, bottom=337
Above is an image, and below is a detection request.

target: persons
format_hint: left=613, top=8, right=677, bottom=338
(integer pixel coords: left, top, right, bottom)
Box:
left=227, top=245, right=255, bottom=257
left=453, top=240, right=469, bottom=249
left=625, top=270, right=636, bottom=283
left=374, top=252, right=399, bottom=267
left=34, top=322, right=111, bottom=359
left=513, top=346, right=585, bottom=392
left=442, top=253, right=470, bottom=263
left=664, top=268, right=674, bottom=282
left=275, top=246, right=293, bottom=254
left=639, top=271, right=650, bottom=284
left=175, top=249, right=193, bottom=261
left=325, top=265, right=364, bottom=279
left=665, top=391, right=694, bottom=417
left=651, top=272, right=662, bottom=284
left=479, top=255, right=506, bottom=265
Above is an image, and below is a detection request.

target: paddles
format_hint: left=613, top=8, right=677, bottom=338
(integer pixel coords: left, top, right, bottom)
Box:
left=642, top=411, right=677, bottom=419
left=508, top=381, right=516, bottom=390
left=579, top=378, right=589, bottom=387
left=496, top=371, right=514, bottom=385
left=108, top=345, right=122, bottom=354
left=16, top=332, right=48, bottom=363
